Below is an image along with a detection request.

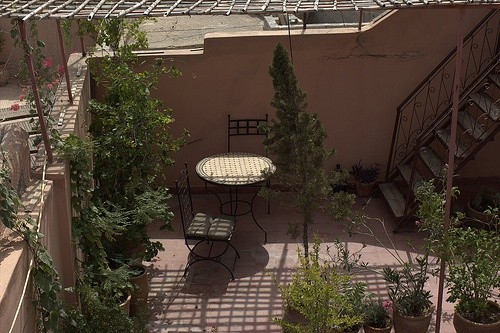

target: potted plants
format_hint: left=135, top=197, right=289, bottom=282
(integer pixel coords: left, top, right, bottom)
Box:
left=466, top=185, right=500, bottom=231
left=413, top=176, right=500, bottom=333
left=363, top=315, right=393, bottom=333
left=385, top=257, right=434, bottom=333
left=280, top=228, right=361, bottom=332
left=70, top=49, right=193, bottom=320
left=347, top=159, right=381, bottom=197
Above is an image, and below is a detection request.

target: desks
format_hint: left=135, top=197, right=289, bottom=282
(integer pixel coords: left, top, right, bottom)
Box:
left=195, top=151, right=276, bottom=244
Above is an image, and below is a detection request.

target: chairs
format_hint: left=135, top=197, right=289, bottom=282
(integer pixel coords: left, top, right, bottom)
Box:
left=227, top=113, right=271, bottom=216
left=174, top=162, right=241, bottom=281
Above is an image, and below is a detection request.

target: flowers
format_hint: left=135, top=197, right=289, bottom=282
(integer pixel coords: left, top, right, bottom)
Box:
left=362, top=299, right=392, bottom=325
